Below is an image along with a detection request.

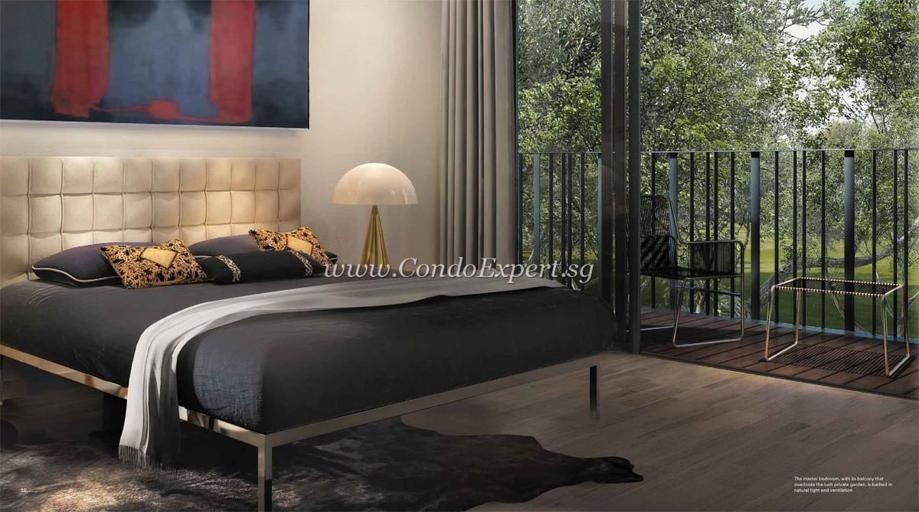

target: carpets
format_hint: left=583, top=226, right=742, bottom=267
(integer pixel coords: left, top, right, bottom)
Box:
left=2, top=418, right=644, bottom=509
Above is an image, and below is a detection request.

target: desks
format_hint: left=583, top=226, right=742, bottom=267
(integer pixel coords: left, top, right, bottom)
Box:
left=763, top=276, right=912, bottom=376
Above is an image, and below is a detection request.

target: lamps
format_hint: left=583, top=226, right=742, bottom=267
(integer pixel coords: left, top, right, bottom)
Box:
left=331, top=162, right=419, bottom=267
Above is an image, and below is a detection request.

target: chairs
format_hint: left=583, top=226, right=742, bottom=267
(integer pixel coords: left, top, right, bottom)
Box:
left=639, top=194, right=745, bottom=347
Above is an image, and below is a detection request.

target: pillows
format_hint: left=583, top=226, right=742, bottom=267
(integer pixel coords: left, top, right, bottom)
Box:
left=31, top=225, right=340, bottom=288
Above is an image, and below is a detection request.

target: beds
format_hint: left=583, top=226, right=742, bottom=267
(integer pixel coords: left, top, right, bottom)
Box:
left=1, top=155, right=597, bottom=505
